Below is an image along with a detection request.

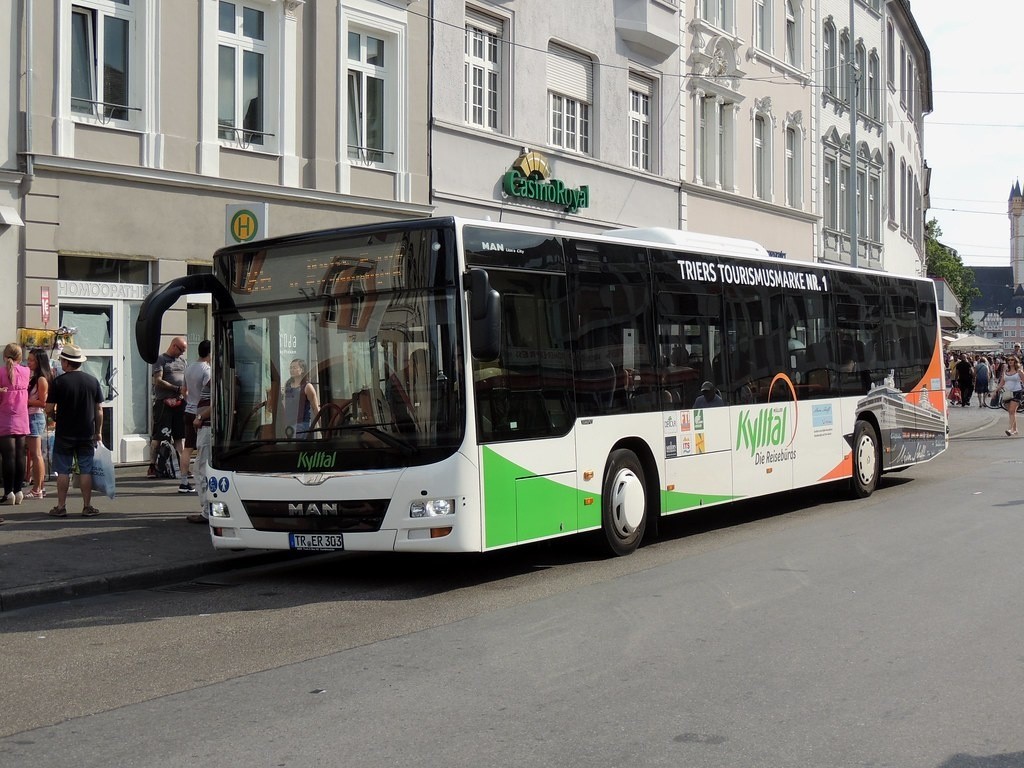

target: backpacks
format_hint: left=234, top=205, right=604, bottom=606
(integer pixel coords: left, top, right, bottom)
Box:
left=154, top=440, right=181, bottom=479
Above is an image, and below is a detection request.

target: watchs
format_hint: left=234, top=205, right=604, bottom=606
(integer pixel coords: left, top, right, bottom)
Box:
left=197, top=414, right=207, bottom=421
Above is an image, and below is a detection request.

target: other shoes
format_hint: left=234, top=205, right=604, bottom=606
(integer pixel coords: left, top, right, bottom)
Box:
left=187, top=514, right=209, bottom=523
left=965, top=402, right=970, bottom=405
left=983, top=403, right=988, bottom=407
left=186, top=470, right=195, bottom=478
left=950, top=401, right=954, bottom=405
left=980, top=405, right=982, bottom=407
left=147, top=467, right=162, bottom=478
left=956, top=402, right=962, bottom=405
left=1005, top=429, right=1017, bottom=436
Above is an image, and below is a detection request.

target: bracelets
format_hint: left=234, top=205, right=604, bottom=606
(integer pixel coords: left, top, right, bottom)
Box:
left=176, top=385, right=182, bottom=393
left=179, top=395, right=185, bottom=401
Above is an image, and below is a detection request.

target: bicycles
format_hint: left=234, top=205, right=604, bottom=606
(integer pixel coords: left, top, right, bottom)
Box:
left=984, top=375, right=1024, bottom=413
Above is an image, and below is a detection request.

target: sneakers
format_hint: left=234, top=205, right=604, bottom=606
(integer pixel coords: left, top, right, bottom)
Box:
left=49, top=505, right=67, bottom=516
left=179, top=483, right=196, bottom=492
left=81, top=507, right=99, bottom=517
left=23, top=487, right=46, bottom=500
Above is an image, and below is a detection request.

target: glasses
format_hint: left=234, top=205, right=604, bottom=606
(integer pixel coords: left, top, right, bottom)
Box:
left=1007, top=359, right=1015, bottom=362
left=175, top=344, right=185, bottom=354
left=1015, top=345, right=1020, bottom=348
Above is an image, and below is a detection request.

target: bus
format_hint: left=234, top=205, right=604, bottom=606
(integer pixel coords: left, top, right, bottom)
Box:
left=135, top=216, right=949, bottom=556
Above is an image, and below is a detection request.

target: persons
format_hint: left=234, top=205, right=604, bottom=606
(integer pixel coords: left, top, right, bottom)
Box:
left=24, top=348, right=54, bottom=499
left=146, top=338, right=194, bottom=479
left=689, top=352, right=702, bottom=367
left=193, top=321, right=280, bottom=445
left=45, top=343, right=104, bottom=517
left=663, top=347, right=704, bottom=409
left=285, top=359, right=321, bottom=440
left=992, top=354, right=1024, bottom=436
left=171, top=340, right=211, bottom=493
left=692, top=382, right=724, bottom=409
left=631, top=360, right=682, bottom=411
left=186, top=379, right=242, bottom=524
left=944, top=342, right=1024, bottom=409
left=0, top=343, right=31, bottom=506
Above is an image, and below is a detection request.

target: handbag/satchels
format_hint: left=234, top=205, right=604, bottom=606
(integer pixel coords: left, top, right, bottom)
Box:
left=988, top=378, right=996, bottom=391
left=1003, top=391, right=1014, bottom=401
left=72, top=440, right=115, bottom=499
left=947, top=384, right=962, bottom=400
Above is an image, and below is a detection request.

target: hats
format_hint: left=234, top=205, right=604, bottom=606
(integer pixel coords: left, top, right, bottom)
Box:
left=700, top=381, right=713, bottom=392
left=58, top=343, right=87, bottom=362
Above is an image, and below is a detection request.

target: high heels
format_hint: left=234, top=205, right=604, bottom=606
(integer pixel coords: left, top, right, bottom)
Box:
left=0, top=492, right=15, bottom=505
left=15, top=491, right=23, bottom=505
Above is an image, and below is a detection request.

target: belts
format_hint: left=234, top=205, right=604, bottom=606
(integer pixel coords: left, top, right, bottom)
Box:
left=202, top=421, right=212, bottom=426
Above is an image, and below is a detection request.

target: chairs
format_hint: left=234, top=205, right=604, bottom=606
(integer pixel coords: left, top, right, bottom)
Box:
left=474, top=329, right=932, bottom=426
